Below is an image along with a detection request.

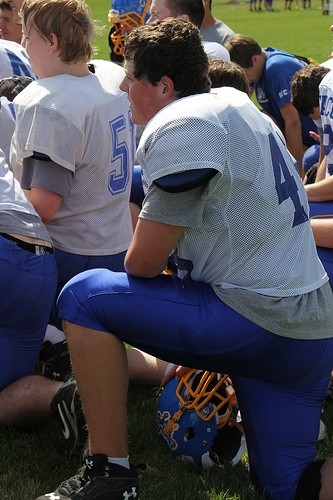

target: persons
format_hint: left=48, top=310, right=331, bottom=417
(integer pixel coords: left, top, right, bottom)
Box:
left=0, top=0, right=333, bottom=458
left=36, top=19, right=333, bottom=500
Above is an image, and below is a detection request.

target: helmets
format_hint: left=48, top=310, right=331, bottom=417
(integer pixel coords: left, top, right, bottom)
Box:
left=154, top=368, right=246, bottom=470
left=112, top=0, right=152, bottom=29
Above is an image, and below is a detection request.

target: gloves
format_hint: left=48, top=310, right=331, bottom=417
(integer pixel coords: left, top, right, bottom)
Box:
left=0, top=75, right=34, bottom=102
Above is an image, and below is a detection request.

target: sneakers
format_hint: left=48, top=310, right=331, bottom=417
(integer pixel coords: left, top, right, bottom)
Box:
left=47, top=378, right=81, bottom=467
left=36, top=449, right=139, bottom=500
